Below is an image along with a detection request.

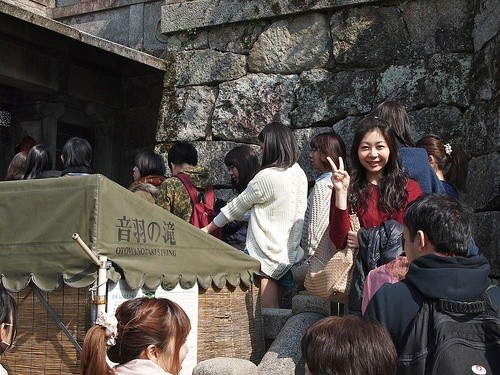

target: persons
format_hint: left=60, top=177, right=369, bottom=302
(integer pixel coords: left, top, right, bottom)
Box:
left=327, top=117, right=425, bottom=315
left=373, top=98, right=447, bottom=197
left=224, top=145, right=262, bottom=249
left=198, top=121, right=308, bottom=308
left=4, top=144, right=51, bottom=181
left=81, top=296, right=191, bottom=375
left=301, top=131, right=346, bottom=261
left=414, top=132, right=459, bottom=197
left=61, top=136, right=94, bottom=176
left=129, top=149, right=167, bottom=205
left=300, top=315, right=399, bottom=375
left=155, top=141, right=216, bottom=223
left=363, top=193, right=500, bottom=346
left=0, top=286, right=18, bottom=375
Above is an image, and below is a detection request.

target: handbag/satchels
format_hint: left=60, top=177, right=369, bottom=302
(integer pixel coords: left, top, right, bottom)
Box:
left=304, top=200, right=362, bottom=303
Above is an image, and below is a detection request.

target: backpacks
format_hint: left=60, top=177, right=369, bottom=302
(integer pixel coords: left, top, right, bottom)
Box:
left=174, top=171, right=222, bottom=240
left=397, top=282, right=500, bottom=375
